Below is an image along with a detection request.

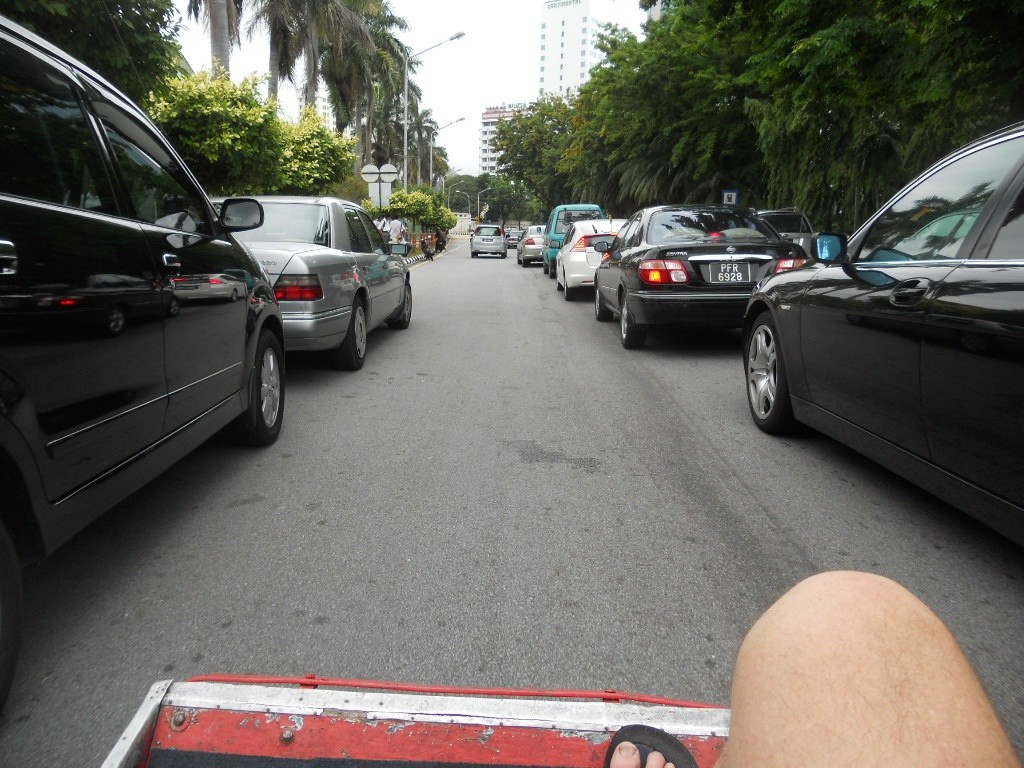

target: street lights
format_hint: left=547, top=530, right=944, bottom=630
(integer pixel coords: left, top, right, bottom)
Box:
left=404, top=32, right=465, bottom=195
left=448, top=180, right=464, bottom=208
left=429, top=117, right=465, bottom=187
left=478, top=188, right=491, bottom=222
left=456, top=191, right=470, bottom=214
left=442, top=169, right=461, bottom=195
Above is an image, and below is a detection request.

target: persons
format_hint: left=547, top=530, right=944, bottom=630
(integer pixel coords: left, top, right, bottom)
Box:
left=603, top=568, right=1022, bottom=767
left=434, top=227, right=447, bottom=252
left=373, top=214, right=412, bottom=258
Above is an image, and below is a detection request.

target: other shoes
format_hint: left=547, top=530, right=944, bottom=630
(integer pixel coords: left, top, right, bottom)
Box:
left=603, top=724, right=699, bottom=768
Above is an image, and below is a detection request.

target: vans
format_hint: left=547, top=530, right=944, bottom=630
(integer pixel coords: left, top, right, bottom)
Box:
left=0, top=13, right=286, bottom=711
left=542, top=205, right=606, bottom=278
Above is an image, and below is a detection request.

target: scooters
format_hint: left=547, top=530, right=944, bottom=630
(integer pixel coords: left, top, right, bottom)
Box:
left=415, top=239, right=435, bottom=261
left=436, top=236, right=447, bottom=252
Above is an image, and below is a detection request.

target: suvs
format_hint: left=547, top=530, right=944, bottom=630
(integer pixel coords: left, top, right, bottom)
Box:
left=749, top=206, right=813, bottom=259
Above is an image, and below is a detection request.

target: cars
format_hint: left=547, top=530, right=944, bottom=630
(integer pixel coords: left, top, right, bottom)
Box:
left=517, top=224, right=547, bottom=267
left=556, top=218, right=628, bottom=301
left=742, top=120, right=1024, bottom=546
left=470, top=225, right=524, bottom=258
left=210, top=196, right=413, bottom=372
left=593, top=205, right=807, bottom=348
